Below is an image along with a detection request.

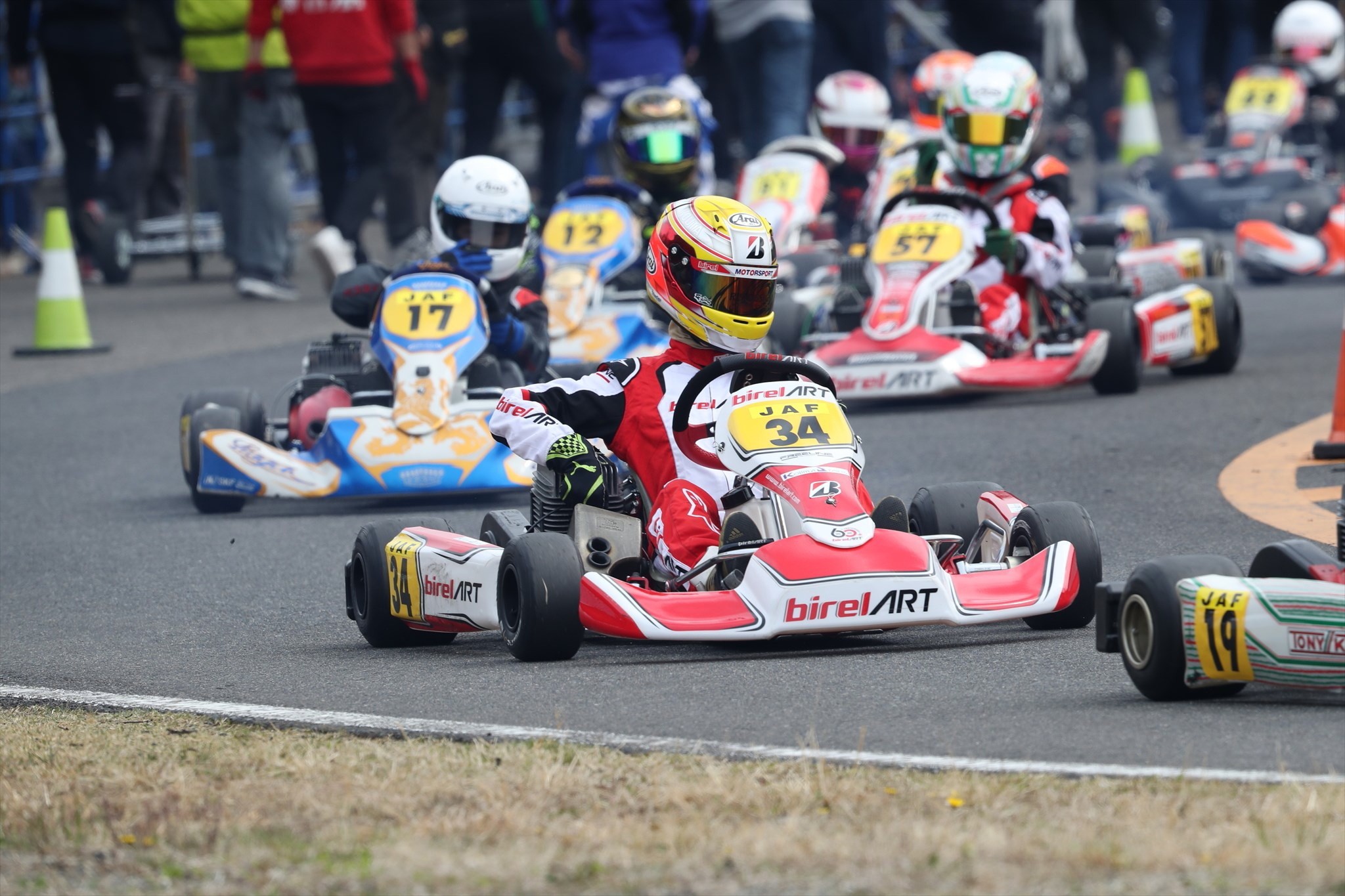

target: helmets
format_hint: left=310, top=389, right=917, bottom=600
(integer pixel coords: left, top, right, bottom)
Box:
left=807, top=71, right=891, bottom=172
left=609, top=86, right=703, bottom=189
left=1273, top=1, right=1345, bottom=87
left=646, top=195, right=779, bottom=355
left=940, top=52, right=1043, bottom=177
left=909, top=50, right=976, bottom=139
left=429, top=155, right=530, bottom=282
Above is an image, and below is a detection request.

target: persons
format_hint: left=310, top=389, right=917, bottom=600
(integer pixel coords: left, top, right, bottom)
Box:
left=489, top=196, right=911, bottom=591
left=0, top=0, right=1345, bottom=364
left=331, top=156, right=548, bottom=407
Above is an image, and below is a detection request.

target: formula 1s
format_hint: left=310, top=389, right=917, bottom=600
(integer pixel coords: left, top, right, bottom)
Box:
left=1092, top=474, right=1344, bottom=705
left=182, top=123, right=1241, bottom=663
left=1130, top=62, right=1345, bottom=286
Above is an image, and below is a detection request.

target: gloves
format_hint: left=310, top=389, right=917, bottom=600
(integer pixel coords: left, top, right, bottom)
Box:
left=1010, top=240, right=1029, bottom=273
left=490, top=313, right=526, bottom=356
left=547, top=433, right=607, bottom=509
left=392, top=239, right=493, bottom=285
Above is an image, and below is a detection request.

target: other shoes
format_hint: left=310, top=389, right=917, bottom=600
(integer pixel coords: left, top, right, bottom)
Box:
left=870, top=496, right=910, bottom=534
left=705, top=511, right=763, bottom=592
left=236, top=275, right=301, bottom=301
left=1169, top=136, right=1209, bottom=164
left=310, top=225, right=357, bottom=294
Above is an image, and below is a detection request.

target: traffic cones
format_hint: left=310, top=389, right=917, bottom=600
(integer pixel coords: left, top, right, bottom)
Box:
left=11, top=207, right=112, bottom=358
left=1119, top=66, right=1164, bottom=170
left=1312, top=317, right=1345, bottom=460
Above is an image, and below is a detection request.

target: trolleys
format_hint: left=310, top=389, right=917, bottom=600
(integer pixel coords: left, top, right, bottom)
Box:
left=80, top=55, right=294, bottom=286
